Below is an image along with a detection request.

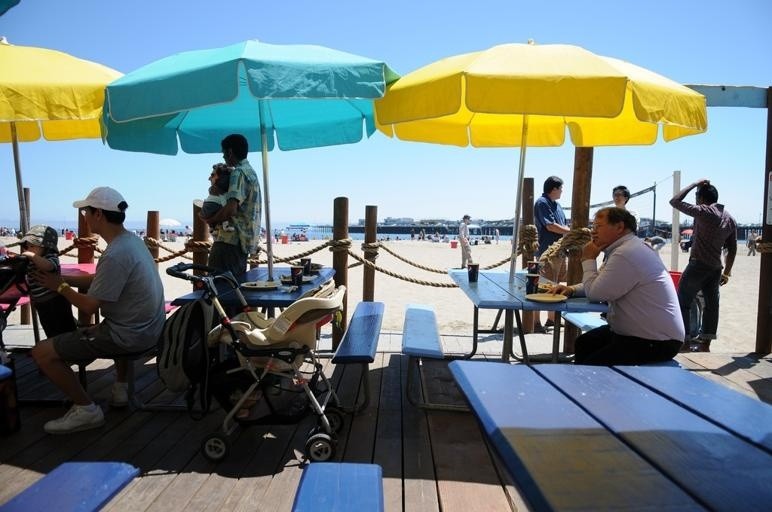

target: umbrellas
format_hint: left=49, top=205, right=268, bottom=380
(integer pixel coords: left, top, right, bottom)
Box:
left=369, top=37, right=709, bottom=363
left=97, top=37, right=402, bottom=282
left=0, top=37, right=126, bottom=238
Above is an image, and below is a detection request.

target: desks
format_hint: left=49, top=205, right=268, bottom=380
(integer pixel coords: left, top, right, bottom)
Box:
left=0, top=263, right=101, bottom=408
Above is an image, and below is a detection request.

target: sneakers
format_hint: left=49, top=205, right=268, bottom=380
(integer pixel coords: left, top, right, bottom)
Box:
left=545, top=318, right=565, bottom=329
left=44, top=403, right=106, bottom=435
left=111, top=381, right=129, bottom=406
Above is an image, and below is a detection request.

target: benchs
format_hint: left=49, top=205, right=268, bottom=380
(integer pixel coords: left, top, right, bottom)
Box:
left=290, top=461, right=386, bottom=510
left=331, top=298, right=480, bottom=412
left=2, top=460, right=142, bottom=512
left=561, top=310, right=680, bottom=367
left=1, top=295, right=39, bottom=352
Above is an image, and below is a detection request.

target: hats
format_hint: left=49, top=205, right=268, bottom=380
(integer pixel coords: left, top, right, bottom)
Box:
left=73, top=186, right=126, bottom=213
left=17, top=224, right=58, bottom=253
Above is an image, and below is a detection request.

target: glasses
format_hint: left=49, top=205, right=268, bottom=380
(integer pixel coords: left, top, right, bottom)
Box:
left=613, top=192, right=624, bottom=197
left=81, top=209, right=87, bottom=217
left=591, top=222, right=608, bottom=231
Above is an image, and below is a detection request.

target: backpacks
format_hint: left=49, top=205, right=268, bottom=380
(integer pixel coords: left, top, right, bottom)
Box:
left=156, top=291, right=214, bottom=398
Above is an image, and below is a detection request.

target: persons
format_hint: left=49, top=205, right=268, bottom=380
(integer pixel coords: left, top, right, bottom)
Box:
left=4, top=226, right=77, bottom=338
left=199, top=165, right=234, bottom=234
left=669, top=178, right=737, bottom=353
left=612, top=186, right=640, bottom=231
left=547, top=207, right=685, bottom=366
left=535, top=175, right=569, bottom=332
left=206, top=134, right=262, bottom=278
left=34, top=186, right=167, bottom=434
left=2, top=215, right=761, bottom=257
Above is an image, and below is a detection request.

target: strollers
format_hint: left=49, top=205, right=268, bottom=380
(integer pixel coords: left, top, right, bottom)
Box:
left=198, top=272, right=349, bottom=463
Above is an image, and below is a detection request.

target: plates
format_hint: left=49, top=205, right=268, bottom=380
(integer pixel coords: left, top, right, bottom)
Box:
left=525, top=293, right=568, bottom=304
left=280, top=276, right=317, bottom=284
left=240, top=281, right=282, bottom=290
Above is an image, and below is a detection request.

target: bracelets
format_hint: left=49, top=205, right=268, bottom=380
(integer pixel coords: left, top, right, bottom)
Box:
left=568, top=285, right=575, bottom=297
left=57, top=282, right=70, bottom=293
left=580, top=257, right=595, bottom=262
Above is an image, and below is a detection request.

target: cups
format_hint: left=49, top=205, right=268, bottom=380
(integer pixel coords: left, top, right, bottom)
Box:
left=300, top=258, right=311, bottom=277
left=527, top=260, right=539, bottom=274
left=467, top=264, right=479, bottom=282
left=525, top=273, right=540, bottom=294
left=290, top=267, right=303, bottom=287
